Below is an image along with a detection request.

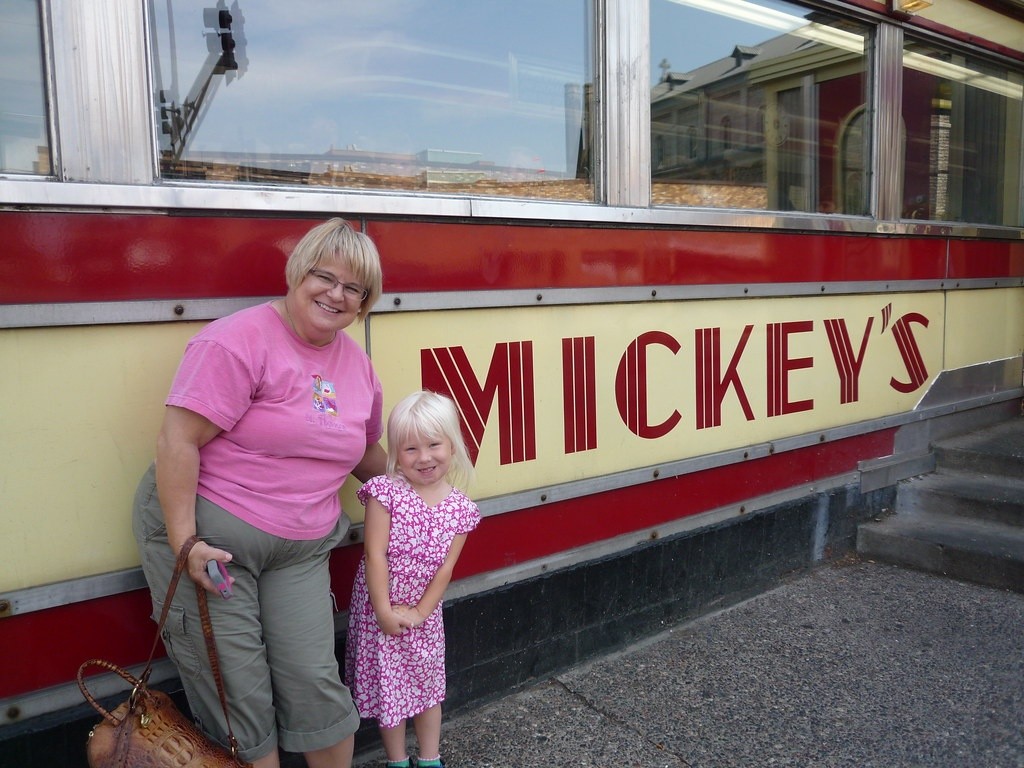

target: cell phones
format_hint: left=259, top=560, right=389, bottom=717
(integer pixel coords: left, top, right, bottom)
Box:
left=207, top=559, right=234, bottom=600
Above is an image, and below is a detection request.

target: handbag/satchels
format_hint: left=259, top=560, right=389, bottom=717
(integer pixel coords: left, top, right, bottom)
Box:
left=76, top=660, right=252, bottom=768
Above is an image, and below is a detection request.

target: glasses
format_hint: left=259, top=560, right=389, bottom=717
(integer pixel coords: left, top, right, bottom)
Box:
left=309, top=270, right=368, bottom=300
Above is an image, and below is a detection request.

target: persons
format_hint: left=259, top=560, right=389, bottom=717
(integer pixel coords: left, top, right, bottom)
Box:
left=345, top=390, right=481, bottom=768
left=132, top=218, right=388, bottom=768
